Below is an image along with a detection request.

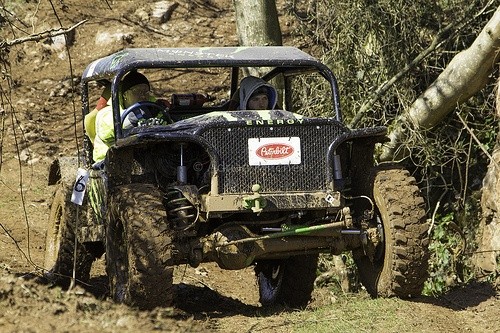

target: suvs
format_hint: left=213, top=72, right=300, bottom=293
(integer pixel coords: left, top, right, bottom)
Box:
left=42, top=46, right=431, bottom=311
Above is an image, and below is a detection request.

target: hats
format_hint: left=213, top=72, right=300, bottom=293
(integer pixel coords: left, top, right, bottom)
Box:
left=121, top=72, right=150, bottom=95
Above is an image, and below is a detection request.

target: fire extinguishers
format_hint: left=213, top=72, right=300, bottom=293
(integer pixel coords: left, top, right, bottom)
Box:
left=172, top=92, right=215, bottom=109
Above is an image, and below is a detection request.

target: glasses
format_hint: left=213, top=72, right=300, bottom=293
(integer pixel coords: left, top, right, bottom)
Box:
left=128, top=88, right=150, bottom=96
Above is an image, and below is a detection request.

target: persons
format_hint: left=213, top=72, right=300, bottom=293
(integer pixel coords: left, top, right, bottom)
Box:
left=83, top=81, right=112, bottom=147
left=92, top=71, right=171, bottom=163
left=236, top=75, right=279, bottom=110
